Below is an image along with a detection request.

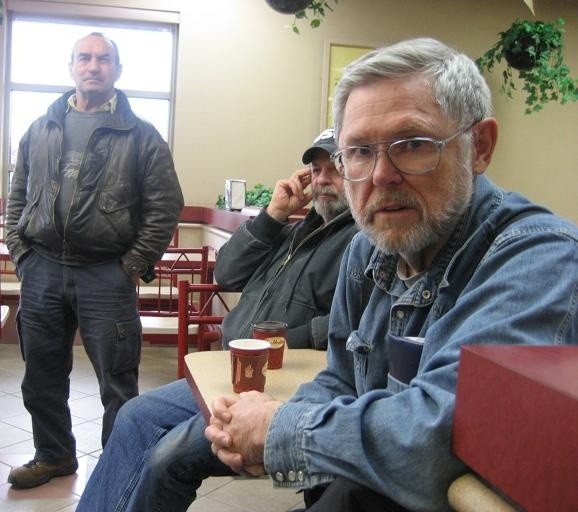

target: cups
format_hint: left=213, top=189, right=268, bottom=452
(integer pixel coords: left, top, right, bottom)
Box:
left=250, top=319, right=289, bottom=370
left=228, top=339, right=272, bottom=394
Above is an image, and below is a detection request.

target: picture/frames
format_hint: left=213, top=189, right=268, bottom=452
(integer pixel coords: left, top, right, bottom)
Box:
left=321, top=38, right=376, bottom=131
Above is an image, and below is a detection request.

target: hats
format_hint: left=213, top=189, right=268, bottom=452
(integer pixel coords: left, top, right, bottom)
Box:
left=302, top=128, right=336, bottom=164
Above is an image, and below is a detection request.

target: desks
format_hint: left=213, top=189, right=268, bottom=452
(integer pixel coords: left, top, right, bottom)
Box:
left=182, top=347, right=327, bottom=427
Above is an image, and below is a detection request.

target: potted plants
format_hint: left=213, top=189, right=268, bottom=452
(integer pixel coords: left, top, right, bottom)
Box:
left=474, top=18, right=577, bottom=116
left=264, top=0, right=339, bottom=34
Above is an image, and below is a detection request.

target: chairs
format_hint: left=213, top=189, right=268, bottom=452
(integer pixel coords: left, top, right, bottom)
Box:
left=137, top=225, right=229, bottom=381
left=0, top=197, right=21, bottom=344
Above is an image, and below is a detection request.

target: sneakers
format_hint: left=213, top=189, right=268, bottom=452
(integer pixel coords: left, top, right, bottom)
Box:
left=8, top=457, right=78, bottom=487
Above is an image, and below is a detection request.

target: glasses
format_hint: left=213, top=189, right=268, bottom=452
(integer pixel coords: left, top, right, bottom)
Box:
left=329, top=119, right=482, bottom=182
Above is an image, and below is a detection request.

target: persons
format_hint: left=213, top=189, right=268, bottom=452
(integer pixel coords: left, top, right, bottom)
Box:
left=203, top=35, right=578, bottom=512
left=73, top=127, right=361, bottom=512
left=4, top=32, right=184, bottom=488
